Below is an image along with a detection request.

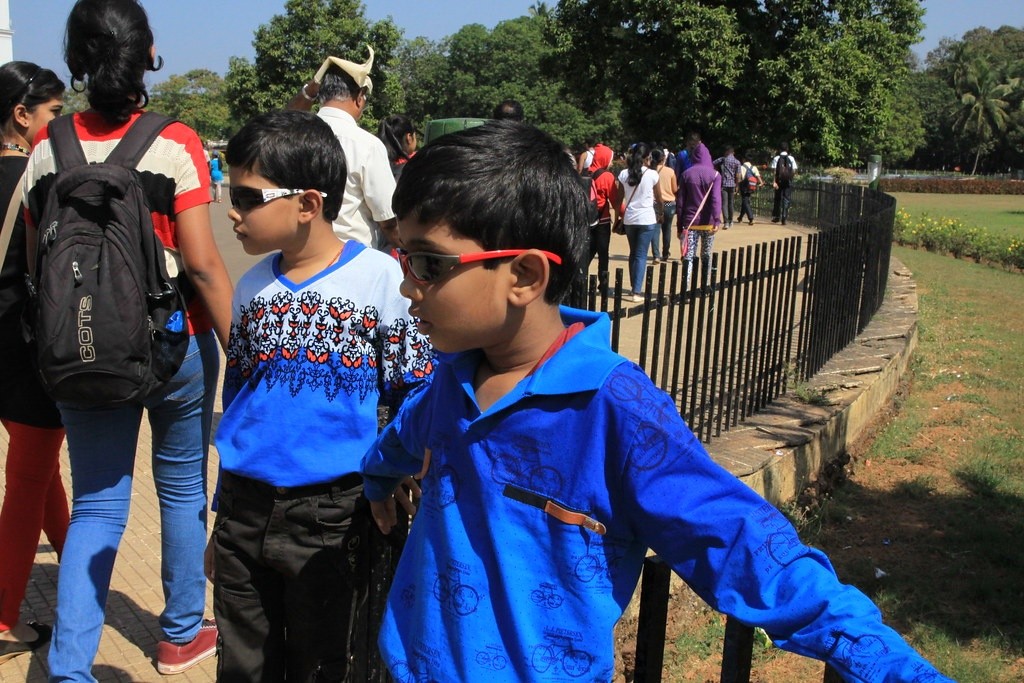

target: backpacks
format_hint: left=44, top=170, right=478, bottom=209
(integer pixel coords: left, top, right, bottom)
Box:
left=740, top=164, right=758, bottom=196
left=775, top=155, right=792, bottom=186
left=21, top=112, right=184, bottom=410
left=579, top=168, right=607, bottom=227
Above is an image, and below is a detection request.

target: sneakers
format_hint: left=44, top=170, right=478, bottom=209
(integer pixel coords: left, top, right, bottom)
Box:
left=157, top=617, right=218, bottom=674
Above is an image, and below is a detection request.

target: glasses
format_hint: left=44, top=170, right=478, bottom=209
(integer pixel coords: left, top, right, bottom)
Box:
left=229, top=185, right=327, bottom=212
left=396, top=248, right=562, bottom=285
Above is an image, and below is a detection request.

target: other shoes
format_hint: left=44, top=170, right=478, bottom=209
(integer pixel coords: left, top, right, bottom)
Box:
left=722, top=225, right=729, bottom=230
left=599, top=289, right=614, bottom=296
left=663, top=253, right=670, bottom=261
left=652, top=258, right=659, bottom=265
left=0, top=620, right=52, bottom=663
left=748, top=220, right=755, bottom=225
left=729, top=223, right=733, bottom=228
left=783, top=220, right=787, bottom=224
left=737, top=217, right=741, bottom=223
left=771, top=218, right=779, bottom=223
left=633, top=295, right=645, bottom=303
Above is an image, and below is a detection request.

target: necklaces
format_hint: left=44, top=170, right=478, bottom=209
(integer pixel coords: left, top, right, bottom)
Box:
left=326, top=246, right=344, bottom=268
left=0, top=143, right=31, bottom=156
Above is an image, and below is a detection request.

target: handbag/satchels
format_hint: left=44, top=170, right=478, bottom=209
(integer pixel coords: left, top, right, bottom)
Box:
left=614, top=219, right=625, bottom=234
left=667, top=151, right=676, bottom=171
left=678, top=230, right=690, bottom=259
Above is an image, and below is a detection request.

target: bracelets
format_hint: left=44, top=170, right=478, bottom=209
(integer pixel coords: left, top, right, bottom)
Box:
left=302, top=84, right=319, bottom=102
left=658, top=212, right=664, bottom=217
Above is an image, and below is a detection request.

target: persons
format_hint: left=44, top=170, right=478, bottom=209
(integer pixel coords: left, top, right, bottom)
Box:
left=614, top=142, right=665, bottom=302
left=208, top=112, right=441, bottom=683
left=646, top=140, right=722, bottom=303
left=734, top=152, right=764, bottom=225
left=712, top=144, right=742, bottom=230
left=577, top=135, right=625, bottom=298
left=283, top=45, right=398, bottom=253
left=364, top=118, right=961, bottom=683
left=492, top=100, right=523, bottom=122
left=200, top=137, right=225, bottom=204
left=0, top=0, right=235, bottom=683
left=771, top=142, right=798, bottom=225
left=377, top=113, right=425, bottom=179
